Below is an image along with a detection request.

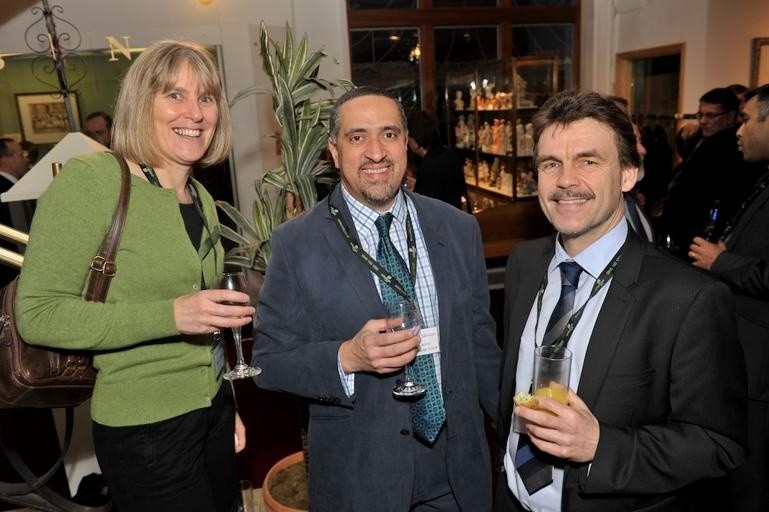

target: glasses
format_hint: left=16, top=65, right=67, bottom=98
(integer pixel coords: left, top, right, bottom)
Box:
left=696, top=108, right=730, bottom=120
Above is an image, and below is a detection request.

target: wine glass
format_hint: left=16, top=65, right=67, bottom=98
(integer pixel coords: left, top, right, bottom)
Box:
left=208, top=274, right=262, bottom=381
left=384, top=300, right=426, bottom=398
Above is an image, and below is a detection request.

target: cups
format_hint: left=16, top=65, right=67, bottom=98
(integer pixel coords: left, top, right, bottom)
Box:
left=533, top=345, right=572, bottom=413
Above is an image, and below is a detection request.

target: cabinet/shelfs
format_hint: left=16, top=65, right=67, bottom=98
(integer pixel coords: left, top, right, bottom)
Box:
left=378, top=51, right=567, bottom=216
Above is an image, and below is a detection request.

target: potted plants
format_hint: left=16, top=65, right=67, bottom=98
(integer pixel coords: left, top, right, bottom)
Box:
left=261, top=422, right=312, bottom=512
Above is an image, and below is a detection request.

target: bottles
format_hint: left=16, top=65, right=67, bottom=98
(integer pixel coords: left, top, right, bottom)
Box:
left=701, top=199, right=722, bottom=242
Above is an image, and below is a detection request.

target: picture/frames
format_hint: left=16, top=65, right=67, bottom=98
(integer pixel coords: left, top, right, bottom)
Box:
left=746, top=34, right=769, bottom=89
left=12, top=86, right=84, bottom=150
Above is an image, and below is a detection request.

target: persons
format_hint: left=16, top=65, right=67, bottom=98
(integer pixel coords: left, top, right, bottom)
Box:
left=492, top=87, right=751, bottom=512
left=10, top=37, right=258, bottom=511
left=247, top=83, right=506, bottom=511
left=607, top=80, right=769, bottom=304
left=402, top=110, right=463, bottom=213
left=0, top=136, right=34, bottom=292
left=81, top=110, right=113, bottom=151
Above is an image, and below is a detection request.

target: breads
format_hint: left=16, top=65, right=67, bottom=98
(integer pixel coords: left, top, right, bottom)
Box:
left=512, top=393, right=539, bottom=409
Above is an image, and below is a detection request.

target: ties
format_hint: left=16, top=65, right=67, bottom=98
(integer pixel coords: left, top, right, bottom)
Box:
left=508, top=258, right=582, bottom=497
left=368, top=214, right=447, bottom=445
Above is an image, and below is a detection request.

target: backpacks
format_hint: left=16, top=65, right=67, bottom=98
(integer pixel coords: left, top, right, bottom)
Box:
left=1, top=268, right=112, bottom=410
